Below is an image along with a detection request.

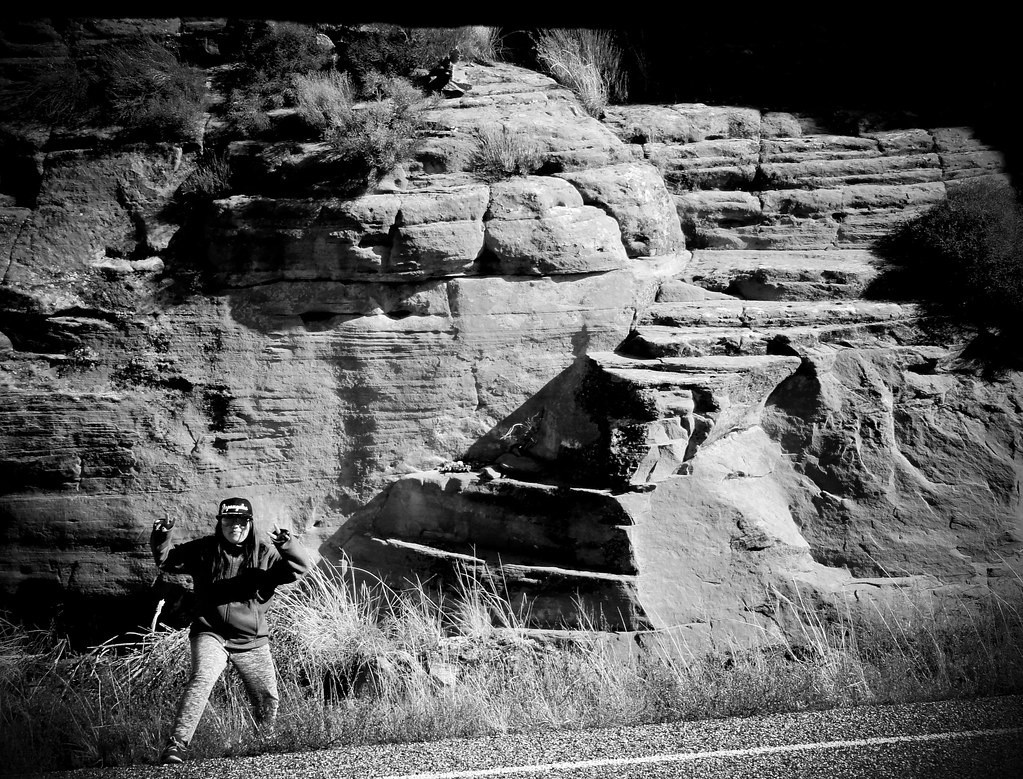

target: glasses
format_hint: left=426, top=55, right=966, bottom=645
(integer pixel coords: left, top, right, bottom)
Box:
left=221, top=515, right=250, bottom=527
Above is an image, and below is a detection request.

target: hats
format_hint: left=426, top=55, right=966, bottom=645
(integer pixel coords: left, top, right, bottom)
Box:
left=218, top=498, right=253, bottom=517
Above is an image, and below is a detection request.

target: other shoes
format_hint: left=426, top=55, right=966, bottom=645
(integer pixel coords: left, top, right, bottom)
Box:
left=162, top=736, right=187, bottom=763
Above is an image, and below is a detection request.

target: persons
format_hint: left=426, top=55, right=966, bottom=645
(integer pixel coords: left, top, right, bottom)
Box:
left=150, top=497, right=310, bottom=762
